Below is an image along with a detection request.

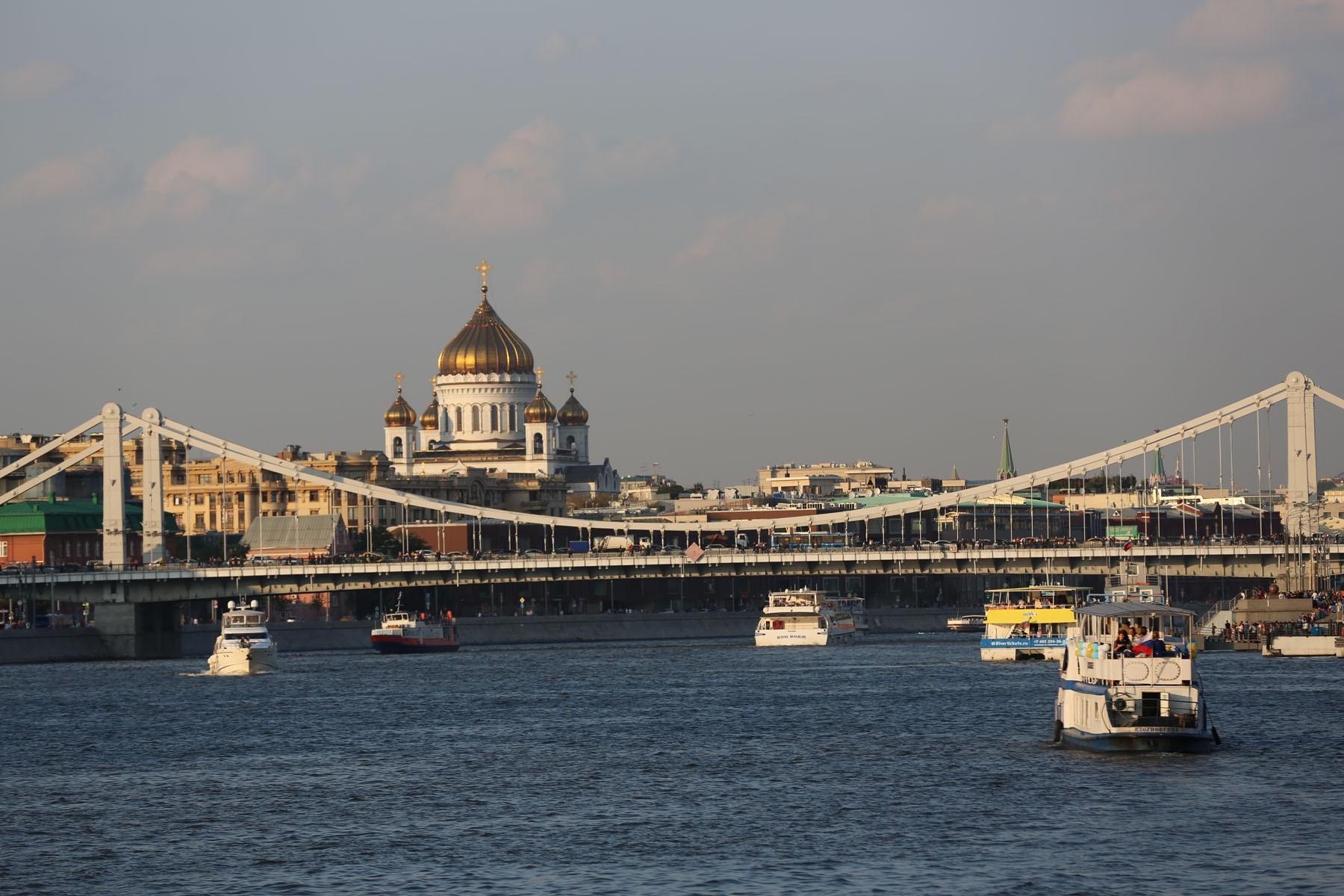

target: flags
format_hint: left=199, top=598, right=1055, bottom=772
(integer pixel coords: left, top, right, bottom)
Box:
left=1123, top=539, right=1133, bottom=551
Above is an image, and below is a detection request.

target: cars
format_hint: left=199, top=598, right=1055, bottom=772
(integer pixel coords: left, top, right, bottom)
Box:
left=1, top=560, right=104, bottom=574
left=921, top=536, right=1039, bottom=549
left=627, top=544, right=684, bottom=555
left=1247, top=533, right=1268, bottom=542
left=655, top=608, right=675, bottom=614
left=1210, top=532, right=1233, bottom=542
left=706, top=544, right=726, bottom=551
left=149, top=554, right=277, bottom=570
left=1052, top=536, right=1105, bottom=547
left=412, top=549, right=470, bottom=562
left=481, top=547, right=569, bottom=560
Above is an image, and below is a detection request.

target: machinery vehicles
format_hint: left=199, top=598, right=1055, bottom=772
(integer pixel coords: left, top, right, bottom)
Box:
left=705, top=532, right=749, bottom=548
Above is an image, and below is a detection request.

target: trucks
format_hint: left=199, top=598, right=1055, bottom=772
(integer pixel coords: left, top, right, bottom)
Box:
left=595, top=535, right=652, bottom=552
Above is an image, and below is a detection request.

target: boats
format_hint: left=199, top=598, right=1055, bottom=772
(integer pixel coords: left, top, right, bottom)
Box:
left=369, top=591, right=461, bottom=655
left=946, top=563, right=1094, bottom=662
left=1054, top=593, right=1219, bottom=754
left=755, top=583, right=871, bottom=647
left=207, top=592, right=278, bottom=677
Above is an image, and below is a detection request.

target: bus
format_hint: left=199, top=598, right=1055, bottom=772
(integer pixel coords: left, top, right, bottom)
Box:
left=768, top=531, right=861, bottom=553
left=34, top=614, right=78, bottom=628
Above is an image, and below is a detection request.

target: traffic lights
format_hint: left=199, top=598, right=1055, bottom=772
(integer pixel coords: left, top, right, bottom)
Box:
left=985, top=593, right=990, bottom=599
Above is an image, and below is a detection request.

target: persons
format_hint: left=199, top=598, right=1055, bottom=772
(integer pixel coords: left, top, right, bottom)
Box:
left=732, top=531, right=1344, bottom=555
left=239, top=636, right=253, bottom=661
left=1110, top=618, right=1181, bottom=658
left=0, top=545, right=483, bottom=580
left=1210, top=584, right=1344, bottom=643
left=618, top=545, right=651, bottom=557
left=986, top=598, right=1098, bottom=639
